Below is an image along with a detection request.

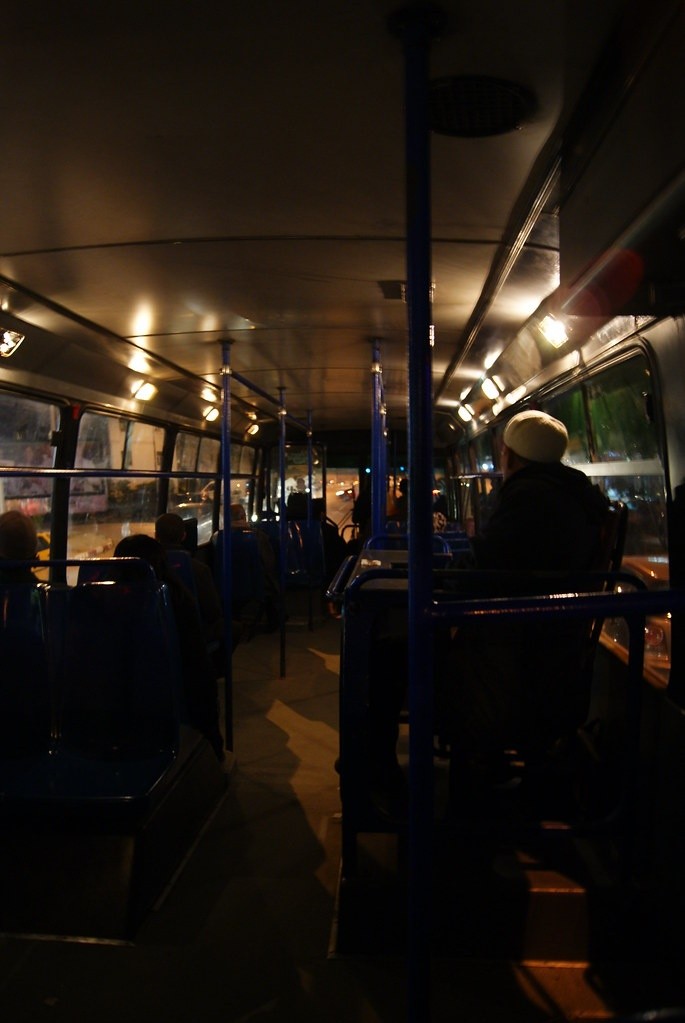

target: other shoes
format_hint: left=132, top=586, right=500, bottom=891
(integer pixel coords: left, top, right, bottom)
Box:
left=267, top=614, right=289, bottom=623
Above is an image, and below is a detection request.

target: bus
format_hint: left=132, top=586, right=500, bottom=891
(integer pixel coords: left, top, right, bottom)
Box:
left=1, top=390, right=117, bottom=515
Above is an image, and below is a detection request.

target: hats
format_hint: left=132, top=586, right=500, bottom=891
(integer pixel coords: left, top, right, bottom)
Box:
left=503, top=409, right=570, bottom=464
left=0, top=512, right=38, bottom=558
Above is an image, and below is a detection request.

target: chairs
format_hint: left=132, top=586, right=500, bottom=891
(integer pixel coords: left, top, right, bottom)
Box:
left=0, top=512, right=473, bottom=820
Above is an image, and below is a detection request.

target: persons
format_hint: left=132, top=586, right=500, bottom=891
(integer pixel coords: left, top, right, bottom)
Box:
left=395, top=478, right=408, bottom=516
left=456, top=410, right=612, bottom=574
left=0, top=509, right=53, bottom=588
left=155, top=513, right=220, bottom=616
left=231, top=504, right=273, bottom=557
left=113, top=534, right=171, bottom=582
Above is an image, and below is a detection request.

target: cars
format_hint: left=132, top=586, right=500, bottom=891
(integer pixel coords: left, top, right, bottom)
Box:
left=178, top=478, right=261, bottom=520
